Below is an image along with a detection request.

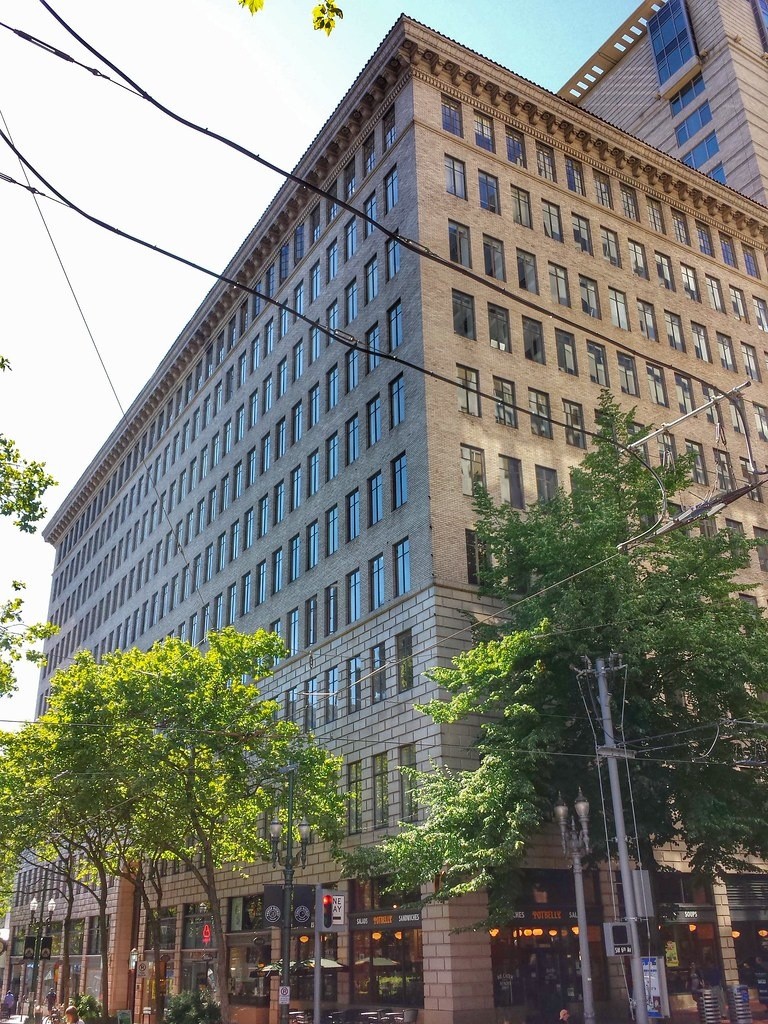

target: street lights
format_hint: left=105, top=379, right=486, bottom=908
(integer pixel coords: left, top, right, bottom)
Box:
left=25, top=897, right=56, bottom=1024
left=553, top=786, right=597, bottom=1024
left=270, top=811, right=311, bottom=1024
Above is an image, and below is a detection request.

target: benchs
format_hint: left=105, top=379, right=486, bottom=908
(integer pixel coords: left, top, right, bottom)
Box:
left=279, top=1009, right=418, bottom=1024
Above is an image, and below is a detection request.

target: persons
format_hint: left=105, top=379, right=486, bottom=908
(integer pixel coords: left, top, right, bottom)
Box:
left=43, top=988, right=57, bottom=1020
left=64, top=1006, right=85, bottom=1024
left=686, top=961, right=705, bottom=1002
left=4, top=989, right=15, bottom=1018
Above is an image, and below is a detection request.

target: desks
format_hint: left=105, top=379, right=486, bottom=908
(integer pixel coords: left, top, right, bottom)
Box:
left=384, top=1013, right=404, bottom=1024
left=361, top=1012, right=378, bottom=1024
left=288, top=1012, right=304, bottom=1024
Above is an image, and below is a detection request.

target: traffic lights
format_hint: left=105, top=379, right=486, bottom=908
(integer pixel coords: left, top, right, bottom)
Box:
left=323, top=895, right=333, bottom=929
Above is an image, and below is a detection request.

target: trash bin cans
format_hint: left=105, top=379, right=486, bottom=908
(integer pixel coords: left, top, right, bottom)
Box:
left=35, top=1006, right=43, bottom=1024
left=727, top=985, right=753, bottom=1024
left=697, top=988, right=721, bottom=1024
